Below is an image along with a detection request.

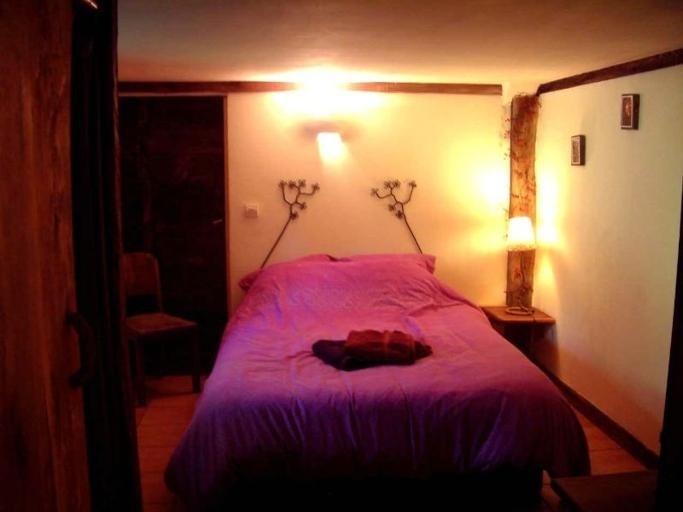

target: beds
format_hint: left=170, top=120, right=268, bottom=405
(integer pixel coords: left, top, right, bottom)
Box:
left=199, top=254, right=559, bottom=511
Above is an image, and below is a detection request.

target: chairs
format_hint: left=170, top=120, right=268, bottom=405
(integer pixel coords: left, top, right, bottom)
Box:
left=122, top=251, right=198, bottom=404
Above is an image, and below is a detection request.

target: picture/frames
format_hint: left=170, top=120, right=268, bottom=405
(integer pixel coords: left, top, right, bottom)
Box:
left=570, top=135, right=585, bottom=165
left=620, top=94, right=636, bottom=130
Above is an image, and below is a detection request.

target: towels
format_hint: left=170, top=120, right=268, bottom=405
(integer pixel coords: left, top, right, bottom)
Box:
left=313, top=329, right=434, bottom=374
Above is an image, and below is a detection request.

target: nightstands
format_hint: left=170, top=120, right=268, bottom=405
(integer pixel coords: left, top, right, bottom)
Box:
left=485, top=305, right=556, bottom=353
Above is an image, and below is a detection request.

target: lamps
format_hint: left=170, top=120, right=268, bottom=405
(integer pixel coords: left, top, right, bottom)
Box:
left=506, top=216, right=535, bottom=316
